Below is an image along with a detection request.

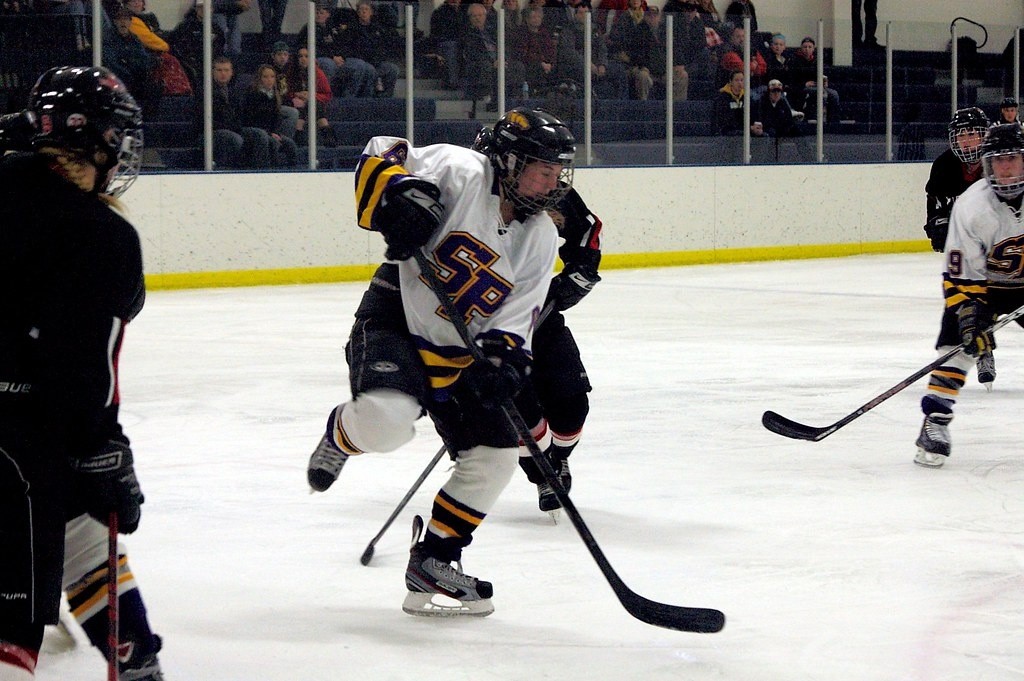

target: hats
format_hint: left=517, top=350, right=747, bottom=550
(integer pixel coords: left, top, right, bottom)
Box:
left=769, top=80, right=783, bottom=91
left=270, top=40, right=290, bottom=51
left=645, top=7, right=659, bottom=15
left=196, top=0, right=205, bottom=6
left=114, top=8, right=129, bottom=16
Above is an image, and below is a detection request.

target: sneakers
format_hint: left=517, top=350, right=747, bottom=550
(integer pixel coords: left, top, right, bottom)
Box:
left=547, top=453, right=572, bottom=495
left=402, top=514, right=495, bottom=619
left=913, top=416, right=951, bottom=466
left=537, top=483, right=563, bottom=525
left=976, top=352, right=995, bottom=393
left=307, top=405, right=350, bottom=495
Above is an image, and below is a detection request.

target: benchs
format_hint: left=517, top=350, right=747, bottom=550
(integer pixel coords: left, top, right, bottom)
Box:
left=137, top=47, right=1012, bottom=173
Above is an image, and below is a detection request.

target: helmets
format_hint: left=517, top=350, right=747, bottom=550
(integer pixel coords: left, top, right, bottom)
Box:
left=948, top=107, right=993, bottom=164
left=491, top=106, right=578, bottom=217
left=24, top=67, right=142, bottom=201
left=1000, top=98, right=1018, bottom=108
left=980, top=121, right=1024, bottom=199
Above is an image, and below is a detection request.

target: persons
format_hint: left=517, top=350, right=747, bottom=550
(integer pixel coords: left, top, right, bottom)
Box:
left=989, top=97, right=1024, bottom=130
left=916, top=122, right=1024, bottom=456
left=0, top=0, right=193, bottom=121
left=170, top=0, right=398, bottom=168
left=923, top=107, right=996, bottom=383
left=472, top=124, right=602, bottom=511
left=0, top=64, right=168, bottom=681
left=657, top=0, right=843, bottom=137
left=852, top=0, right=887, bottom=49
left=307, top=107, right=576, bottom=600
left=427, top=0, right=687, bottom=102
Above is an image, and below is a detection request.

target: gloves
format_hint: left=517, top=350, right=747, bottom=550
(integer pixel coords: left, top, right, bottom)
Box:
left=549, top=263, right=601, bottom=312
left=924, top=212, right=950, bottom=254
left=372, top=175, right=444, bottom=262
left=957, top=306, right=997, bottom=357
left=479, top=328, right=528, bottom=410
left=74, top=434, right=144, bottom=535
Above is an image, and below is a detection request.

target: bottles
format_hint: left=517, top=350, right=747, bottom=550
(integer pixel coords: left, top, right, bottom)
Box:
left=522, top=81, right=529, bottom=100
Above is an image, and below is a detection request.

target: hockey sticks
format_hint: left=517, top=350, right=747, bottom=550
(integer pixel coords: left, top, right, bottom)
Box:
left=415, top=248, right=727, bottom=634
left=359, top=444, right=445, bottom=567
left=105, top=511, right=120, bottom=681
left=760, top=309, right=1019, bottom=443
